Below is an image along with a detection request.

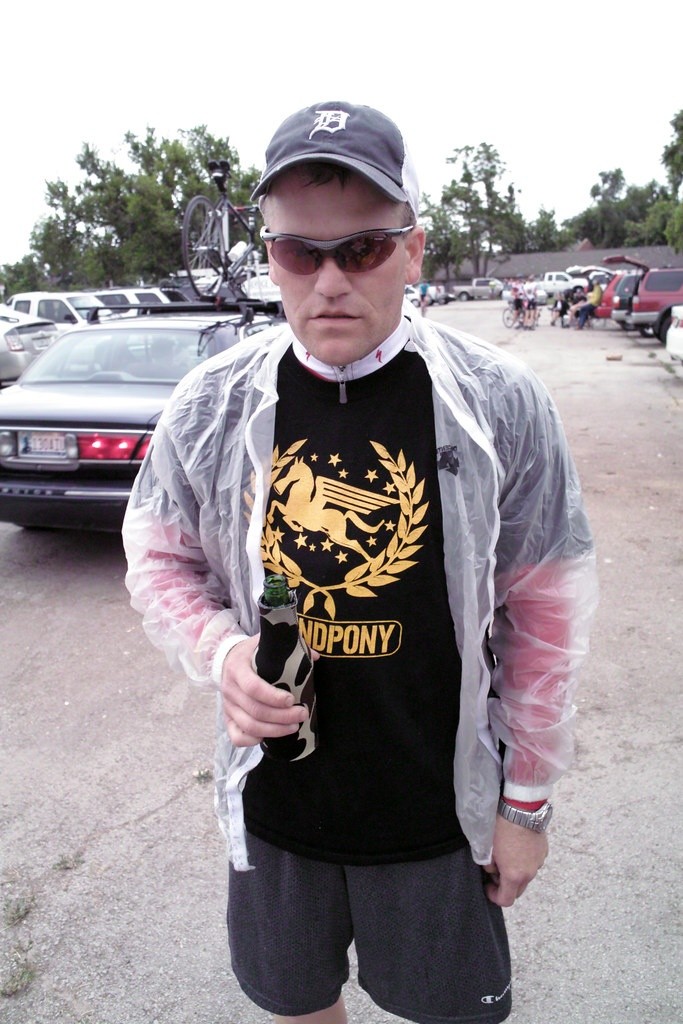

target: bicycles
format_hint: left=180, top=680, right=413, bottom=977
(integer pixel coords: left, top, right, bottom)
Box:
left=502, top=307, right=542, bottom=328
left=182, top=159, right=263, bottom=300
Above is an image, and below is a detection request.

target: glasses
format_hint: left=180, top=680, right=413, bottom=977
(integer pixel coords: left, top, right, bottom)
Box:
left=259, top=225, right=413, bottom=276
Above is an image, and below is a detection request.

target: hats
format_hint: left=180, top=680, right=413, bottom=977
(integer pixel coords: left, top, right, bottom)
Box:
left=248, top=102, right=418, bottom=223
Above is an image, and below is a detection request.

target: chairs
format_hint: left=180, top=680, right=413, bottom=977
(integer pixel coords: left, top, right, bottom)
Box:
left=95, top=340, right=133, bottom=372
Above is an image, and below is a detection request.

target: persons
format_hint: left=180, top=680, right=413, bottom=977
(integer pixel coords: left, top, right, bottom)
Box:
left=120, top=102, right=597, bottom=1024
left=418, top=279, right=430, bottom=317
left=502, top=273, right=602, bottom=331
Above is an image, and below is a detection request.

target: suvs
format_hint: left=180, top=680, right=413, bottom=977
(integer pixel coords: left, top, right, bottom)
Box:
left=0, top=263, right=287, bottom=534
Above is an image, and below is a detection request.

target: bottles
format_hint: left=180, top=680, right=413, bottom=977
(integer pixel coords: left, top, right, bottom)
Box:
left=247, top=574, right=319, bottom=762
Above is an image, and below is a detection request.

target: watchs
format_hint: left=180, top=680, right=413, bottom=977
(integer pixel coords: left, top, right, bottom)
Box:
left=498, top=800, right=553, bottom=832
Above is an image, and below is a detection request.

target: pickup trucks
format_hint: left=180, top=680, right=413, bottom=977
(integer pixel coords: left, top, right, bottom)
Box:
left=422, top=285, right=447, bottom=307
left=501, top=271, right=589, bottom=305
left=452, top=277, right=505, bottom=302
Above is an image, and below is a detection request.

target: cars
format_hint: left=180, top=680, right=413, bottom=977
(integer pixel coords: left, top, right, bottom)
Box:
left=404, top=285, right=421, bottom=308
left=567, top=255, right=683, bottom=380
